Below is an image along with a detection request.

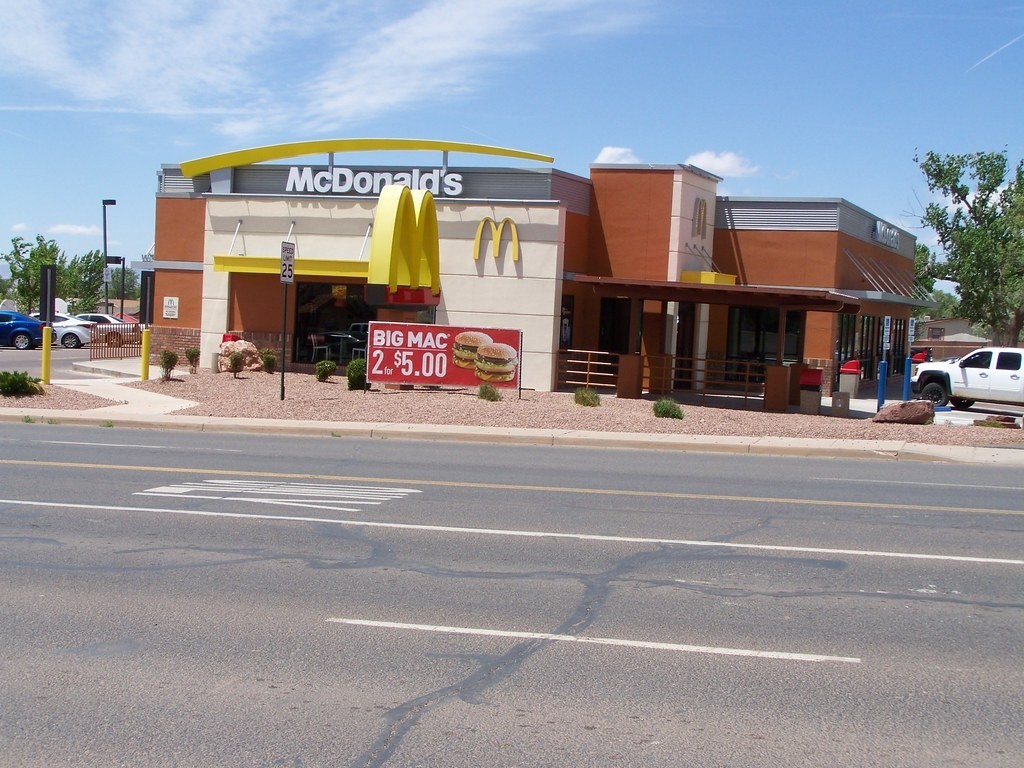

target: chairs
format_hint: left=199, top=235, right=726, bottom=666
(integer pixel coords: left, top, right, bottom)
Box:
left=311, top=334, right=331, bottom=363
left=352, top=342, right=368, bottom=361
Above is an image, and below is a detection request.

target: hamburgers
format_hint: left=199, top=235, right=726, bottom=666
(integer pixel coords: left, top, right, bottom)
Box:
left=474, top=343, right=518, bottom=381
left=452, top=332, right=493, bottom=369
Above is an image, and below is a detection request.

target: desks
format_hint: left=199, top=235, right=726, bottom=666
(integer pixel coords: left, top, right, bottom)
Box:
left=330, top=334, right=352, bottom=365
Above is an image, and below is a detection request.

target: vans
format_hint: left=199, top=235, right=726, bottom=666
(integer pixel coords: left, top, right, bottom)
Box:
left=348, top=323, right=369, bottom=336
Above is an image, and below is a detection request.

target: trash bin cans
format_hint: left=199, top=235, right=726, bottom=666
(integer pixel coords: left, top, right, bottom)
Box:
left=219, top=334, right=241, bottom=348
left=910, top=353, right=925, bottom=381
left=838, top=360, right=862, bottom=399
left=798, top=368, right=822, bottom=415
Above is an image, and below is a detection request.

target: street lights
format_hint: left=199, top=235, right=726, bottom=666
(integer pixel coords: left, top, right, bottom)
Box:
left=102, top=200, right=116, bottom=312
left=107, top=255, right=125, bottom=320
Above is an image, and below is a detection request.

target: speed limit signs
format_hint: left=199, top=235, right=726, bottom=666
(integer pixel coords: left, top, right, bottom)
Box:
left=280, top=241, right=296, bottom=284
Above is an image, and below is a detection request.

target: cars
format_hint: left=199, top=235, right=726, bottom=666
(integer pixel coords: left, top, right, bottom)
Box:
left=308, top=332, right=367, bottom=360
left=29, top=312, right=98, bottom=348
left=910, top=346, right=932, bottom=364
left=75, top=314, right=141, bottom=347
left=0, top=310, right=58, bottom=351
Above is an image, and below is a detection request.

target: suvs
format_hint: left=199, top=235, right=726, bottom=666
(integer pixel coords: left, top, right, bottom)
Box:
left=911, top=345, right=1024, bottom=410
